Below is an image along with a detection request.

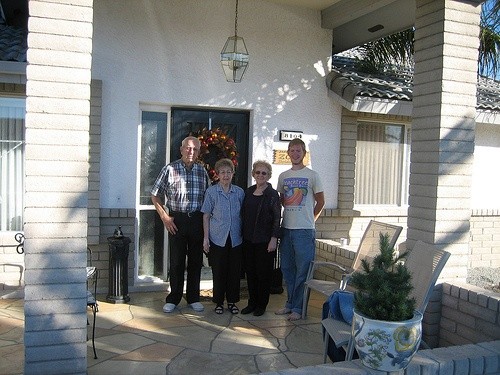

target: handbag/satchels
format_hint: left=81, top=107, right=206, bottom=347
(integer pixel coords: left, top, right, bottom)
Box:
left=262, top=268, right=284, bottom=294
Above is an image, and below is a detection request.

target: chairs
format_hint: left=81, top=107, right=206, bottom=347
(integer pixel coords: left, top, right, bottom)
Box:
left=324, top=240, right=450, bottom=365
left=300, top=220, right=403, bottom=319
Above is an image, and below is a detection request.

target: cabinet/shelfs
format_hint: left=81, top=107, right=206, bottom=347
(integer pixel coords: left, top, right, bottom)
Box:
left=87, top=247, right=99, bottom=359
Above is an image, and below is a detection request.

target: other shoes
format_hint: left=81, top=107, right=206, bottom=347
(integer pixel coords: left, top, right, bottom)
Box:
left=288, top=312, right=302, bottom=321
left=191, top=302, right=204, bottom=312
left=241, top=306, right=253, bottom=316
left=163, top=303, right=175, bottom=313
left=253, top=306, right=267, bottom=316
left=275, top=308, right=291, bottom=315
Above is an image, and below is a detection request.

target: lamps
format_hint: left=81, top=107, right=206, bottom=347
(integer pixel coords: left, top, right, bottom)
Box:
left=220, top=0, right=250, bottom=83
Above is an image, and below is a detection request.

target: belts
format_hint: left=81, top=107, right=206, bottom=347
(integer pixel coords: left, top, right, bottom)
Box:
left=170, top=209, right=202, bottom=218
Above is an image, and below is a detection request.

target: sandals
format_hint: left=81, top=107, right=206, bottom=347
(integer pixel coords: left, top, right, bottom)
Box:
left=215, top=304, right=224, bottom=313
left=228, top=303, right=240, bottom=314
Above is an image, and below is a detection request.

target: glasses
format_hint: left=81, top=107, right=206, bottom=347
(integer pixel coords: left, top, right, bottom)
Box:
left=254, top=171, right=267, bottom=175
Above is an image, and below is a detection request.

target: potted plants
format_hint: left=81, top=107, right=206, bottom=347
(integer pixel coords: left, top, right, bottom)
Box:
left=344, top=231, right=424, bottom=372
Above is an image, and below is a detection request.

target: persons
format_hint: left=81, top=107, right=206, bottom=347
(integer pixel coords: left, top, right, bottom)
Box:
left=276, top=138, right=325, bottom=319
left=149, top=136, right=212, bottom=313
left=200, top=159, right=245, bottom=315
left=239, top=161, right=281, bottom=317
